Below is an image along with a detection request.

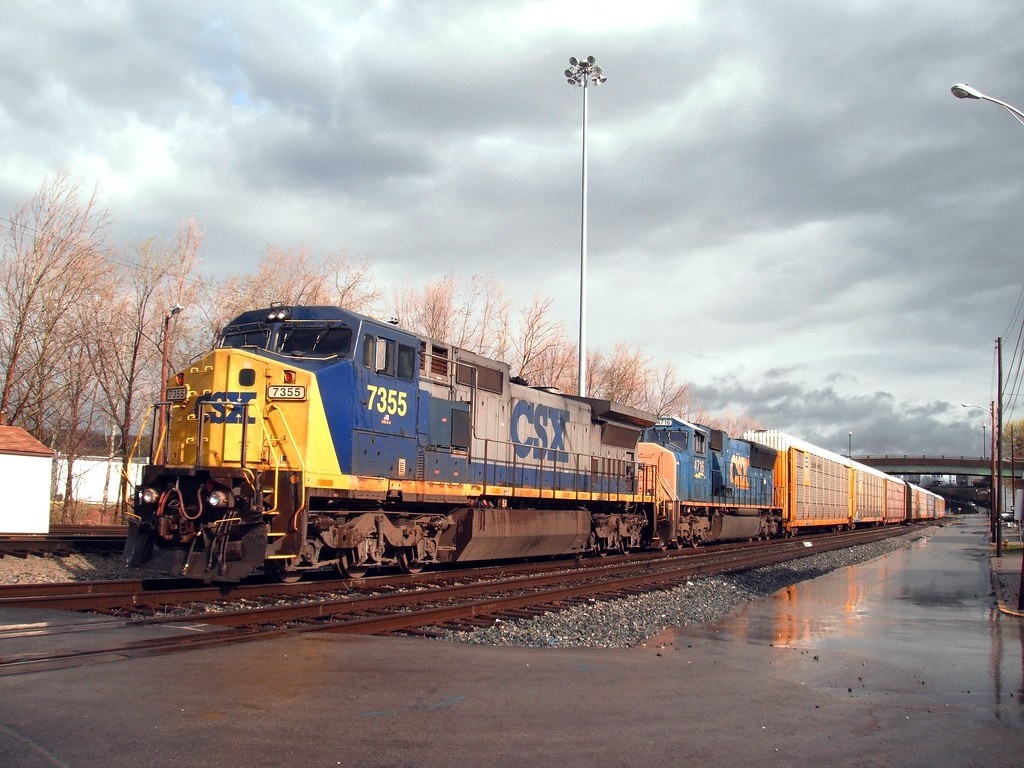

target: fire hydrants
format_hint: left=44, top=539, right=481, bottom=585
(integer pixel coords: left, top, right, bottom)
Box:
left=1002, top=539, right=1009, bottom=552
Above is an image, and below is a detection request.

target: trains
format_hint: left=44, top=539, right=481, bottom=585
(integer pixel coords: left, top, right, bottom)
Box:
left=121, top=301, right=945, bottom=584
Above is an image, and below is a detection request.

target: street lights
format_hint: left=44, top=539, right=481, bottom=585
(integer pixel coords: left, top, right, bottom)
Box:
left=849, top=430, right=852, bottom=456
left=960, top=401, right=995, bottom=541
left=982, top=423, right=986, bottom=459
left=157, top=303, right=185, bottom=465
left=563, top=56, right=608, bottom=400
